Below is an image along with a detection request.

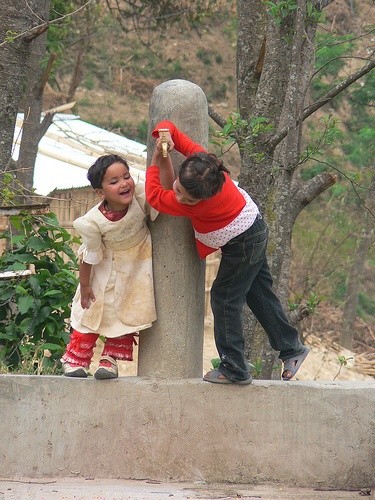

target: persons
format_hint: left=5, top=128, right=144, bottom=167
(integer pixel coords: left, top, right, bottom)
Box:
left=144, top=120, right=309, bottom=384
left=60, top=137, right=175, bottom=379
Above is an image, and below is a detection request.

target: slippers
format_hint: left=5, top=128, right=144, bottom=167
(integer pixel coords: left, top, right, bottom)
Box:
left=203, top=368, right=253, bottom=385
left=281, top=346, right=310, bottom=379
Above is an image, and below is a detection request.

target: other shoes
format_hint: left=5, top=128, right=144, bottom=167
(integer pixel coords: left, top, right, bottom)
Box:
left=63, top=363, right=87, bottom=377
left=95, top=356, right=118, bottom=379
